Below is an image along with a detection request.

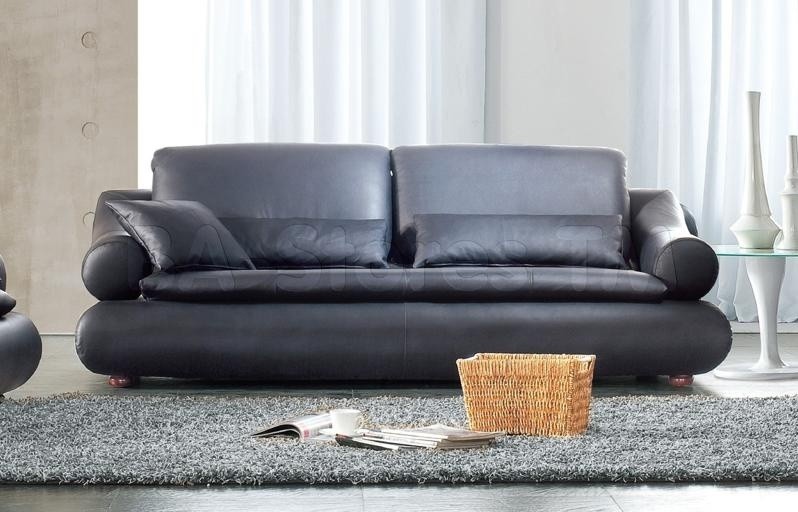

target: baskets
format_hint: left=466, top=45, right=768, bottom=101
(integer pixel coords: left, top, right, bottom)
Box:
left=454, top=348, right=597, bottom=436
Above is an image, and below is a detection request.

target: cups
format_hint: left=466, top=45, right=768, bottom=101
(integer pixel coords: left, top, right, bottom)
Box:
left=328, top=409, right=363, bottom=431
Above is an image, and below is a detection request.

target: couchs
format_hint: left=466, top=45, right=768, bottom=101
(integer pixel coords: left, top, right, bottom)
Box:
left=74, top=145, right=734, bottom=388
left=1, top=258, right=43, bottom=396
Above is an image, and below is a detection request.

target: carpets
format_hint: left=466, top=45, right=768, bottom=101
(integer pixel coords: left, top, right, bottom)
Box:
left=0, top=394, right=798, bottom=484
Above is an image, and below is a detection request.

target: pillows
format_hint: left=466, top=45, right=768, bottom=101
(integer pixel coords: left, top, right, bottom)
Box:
left=107, top=199, right=257, bottom=271
left=219, top=221, right=392, bottom=271
left=414, top=212, right=626, bottom=271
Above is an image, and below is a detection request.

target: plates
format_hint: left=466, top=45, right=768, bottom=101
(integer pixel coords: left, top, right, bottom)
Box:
left=319, top=428, right=368, bottom=437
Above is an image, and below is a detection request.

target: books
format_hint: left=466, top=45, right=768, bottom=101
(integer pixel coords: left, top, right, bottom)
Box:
left=250, top=413, right=362, bottom=444
left=335, top=423, right=504, bottom=454
